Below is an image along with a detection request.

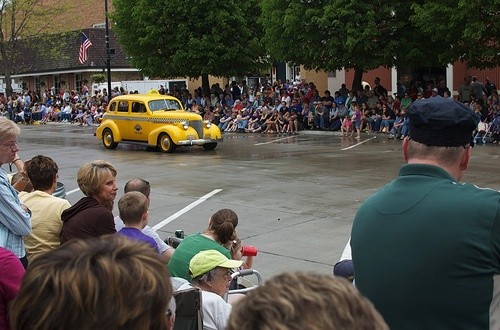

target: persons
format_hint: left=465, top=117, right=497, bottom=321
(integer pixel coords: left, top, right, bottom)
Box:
left=11, top=233, right=173, bottom=330
left=230, top=237, right=253, bottom=274
left=167, top=209, right=246, bottom=305
left=0, top=116, right=32, bottom=270
left=19, top=154, right=72, bottom=265
left=164, top=277, right=190, bottom=330
left=225, top=259, right=391, bottom=330
left=114, top=178, right=176, bottom=265
left=116, top=191, right=161, bottom=255
left=176, top=249, right=243, bottom=330
left=0, top=247, right=27, bottom=330
left=0, top=72, right=500, bottom=149
left=350, top=96, right=500, bottom=330
left=0, top=148, right=35, bottom=197
left=60, top=160, right=117, bottom=245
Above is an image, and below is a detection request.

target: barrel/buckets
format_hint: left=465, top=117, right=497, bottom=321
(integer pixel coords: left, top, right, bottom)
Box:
left=52, top=182, right=66, bottom=199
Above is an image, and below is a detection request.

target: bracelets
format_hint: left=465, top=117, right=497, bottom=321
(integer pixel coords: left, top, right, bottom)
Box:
left=21, top=177, right=30, bottom=183
left=10, top=158, right=20, bottom=166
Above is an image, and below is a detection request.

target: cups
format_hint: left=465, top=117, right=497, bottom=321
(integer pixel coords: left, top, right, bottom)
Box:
left=242, top=246, right=257, bottom=256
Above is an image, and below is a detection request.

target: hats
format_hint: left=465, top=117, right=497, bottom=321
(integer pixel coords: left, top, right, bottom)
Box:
left=334, top=259, right=353, bottom=279
left=406, top=96, right=480, bottom=147
left=188, top=249, right=244, bottom=279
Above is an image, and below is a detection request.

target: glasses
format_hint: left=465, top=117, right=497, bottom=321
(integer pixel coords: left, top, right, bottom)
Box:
left=0, top=140, right=18, bottom=148
left=211, top=271, right=230, bottom=280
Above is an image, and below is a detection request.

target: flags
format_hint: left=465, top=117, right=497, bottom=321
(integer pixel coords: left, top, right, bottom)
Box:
left=79, top=32, right=92, bottom=64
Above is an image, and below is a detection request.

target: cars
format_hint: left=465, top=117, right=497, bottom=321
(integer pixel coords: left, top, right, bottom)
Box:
left=93, top=88, right=222, bottom=154
